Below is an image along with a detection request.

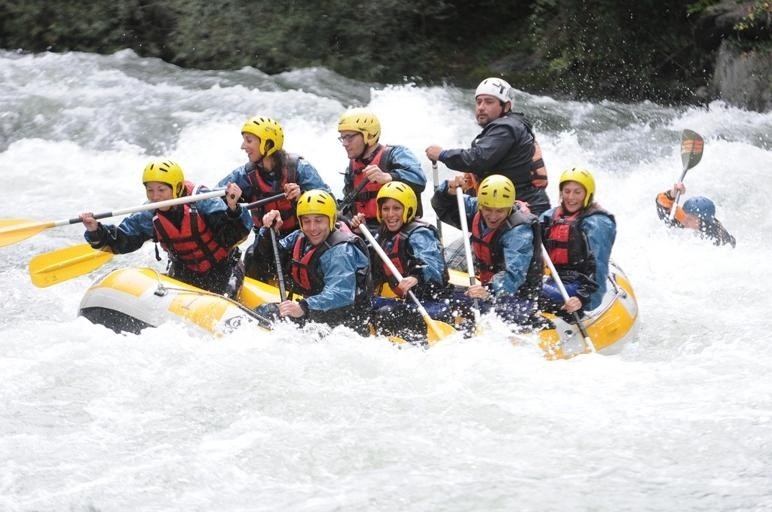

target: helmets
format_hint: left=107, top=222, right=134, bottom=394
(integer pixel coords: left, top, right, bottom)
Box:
left=475, top=77, right=514, bottom=110
left=338, top=111, right=381, bottom=147
left=559, top=167, right=595, bottom=207
left=478, top=174, right=515, bottom=216
left=241, top=115, right=284, bottom=157
left=376, top=181, right=418, bottom=224
left=683, top=196, right=715, bottom=221
left=142, top=159, right=185, bottom=199
left=298, top=189, right=336, bottom=232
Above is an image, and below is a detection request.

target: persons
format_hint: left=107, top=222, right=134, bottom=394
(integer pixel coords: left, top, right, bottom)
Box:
left=430, top=173, right=545, bottom=324
left=81, top=160, right=253, bottom=294
left=656, top=183, right=737, bottom=249
left=350, top=181, right=449, bottom=307
left=210, top=116, right=330, bottom=280
left=514, top=169, right=616, bottom=324
left=424, top=75, right=551, bottom=233
left=333, top=112, right=427, bottom=229
left=244, top=189, right=372, bottom=319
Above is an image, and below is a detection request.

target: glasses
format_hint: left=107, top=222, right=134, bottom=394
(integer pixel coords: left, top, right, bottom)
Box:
left=338, top=132, right=361, bottom=142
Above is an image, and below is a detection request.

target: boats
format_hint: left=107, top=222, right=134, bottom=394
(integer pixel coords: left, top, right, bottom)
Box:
left=78, top=258, right=641, bottom=368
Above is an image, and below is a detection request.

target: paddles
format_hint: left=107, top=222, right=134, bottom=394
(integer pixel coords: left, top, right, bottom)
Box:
left=359, top=220, right=453, bottom=343
left=0, top=189, right=227, bottom=247
left=670, top=128, right=704, bottom=225
left=30, top=193, right=285, bottom=288
